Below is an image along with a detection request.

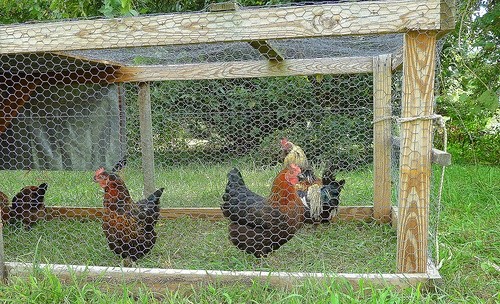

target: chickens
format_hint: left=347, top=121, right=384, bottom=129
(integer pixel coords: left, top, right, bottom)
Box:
left=282, top=138, right=346, bottom=236
left=10, top=183, right=48, bottom=231
left=90, top=168, right=165, bottom=267
left=221, top=163, right=305, bottom=271
